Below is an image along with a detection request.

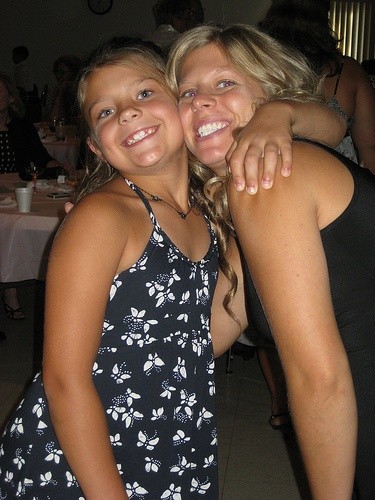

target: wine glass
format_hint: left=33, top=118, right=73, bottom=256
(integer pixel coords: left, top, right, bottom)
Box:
left=26, top=161, right=45, bottom=193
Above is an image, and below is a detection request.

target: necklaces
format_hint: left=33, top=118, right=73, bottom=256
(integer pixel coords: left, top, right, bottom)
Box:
left=135, top=184, right=194, bottom=219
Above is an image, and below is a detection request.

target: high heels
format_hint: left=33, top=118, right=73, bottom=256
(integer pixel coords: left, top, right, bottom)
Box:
left=225, top=340, right=257, bottom=374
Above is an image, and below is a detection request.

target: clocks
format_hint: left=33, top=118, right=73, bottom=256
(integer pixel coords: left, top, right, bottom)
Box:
left=88, top=0, right=113, bottom=15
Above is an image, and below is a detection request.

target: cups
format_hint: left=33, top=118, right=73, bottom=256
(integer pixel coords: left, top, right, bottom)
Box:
left=15, top=188, right=33, bottom=213
left=50, top=116, right=56, bottom=129
left=57, top=121, right=64, bottom=134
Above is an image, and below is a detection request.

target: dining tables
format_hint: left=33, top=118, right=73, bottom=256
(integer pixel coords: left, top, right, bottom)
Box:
left=0, top=172, right=76, bottom=283
left=34, top=122, right=82, bottom=170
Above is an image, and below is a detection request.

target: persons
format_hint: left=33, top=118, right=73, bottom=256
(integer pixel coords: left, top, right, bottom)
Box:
left=0, top=0, right=375, bottom=500
left=0, top=34, right=347, bottom=500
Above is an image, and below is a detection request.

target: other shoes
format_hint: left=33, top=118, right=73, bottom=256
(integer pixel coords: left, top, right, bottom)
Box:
left=5, top=303, right=25, bottom=320
left=268, top=413, right=291, bottom=430
